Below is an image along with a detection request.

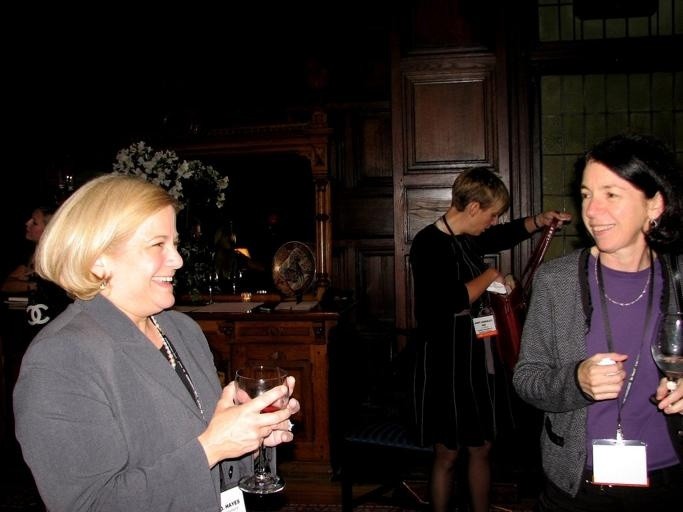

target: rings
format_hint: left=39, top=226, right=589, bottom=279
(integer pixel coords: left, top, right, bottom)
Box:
left=287, top=418, right=295, bottom=432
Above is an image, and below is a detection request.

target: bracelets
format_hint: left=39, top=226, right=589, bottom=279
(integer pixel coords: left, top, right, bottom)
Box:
left=533, top=215, right=541, bottom=230
left=26, top=282, right=31, bottom=292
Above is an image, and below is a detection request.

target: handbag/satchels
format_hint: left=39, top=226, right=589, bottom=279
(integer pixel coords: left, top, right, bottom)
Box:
left=490, top=279, right=524, bottom=366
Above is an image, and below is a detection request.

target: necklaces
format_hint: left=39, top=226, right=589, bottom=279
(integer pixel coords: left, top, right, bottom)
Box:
left=148, top=316, right=177, bottom=370
left=594, top=252, right=651, bottom=306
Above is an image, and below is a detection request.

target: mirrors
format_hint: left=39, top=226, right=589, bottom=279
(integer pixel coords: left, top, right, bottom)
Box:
left=162, top=110, right=333, bottom=302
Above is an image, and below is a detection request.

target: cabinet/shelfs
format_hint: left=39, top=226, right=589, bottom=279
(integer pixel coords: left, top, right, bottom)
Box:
left=148, top=311, right=343, bottom=480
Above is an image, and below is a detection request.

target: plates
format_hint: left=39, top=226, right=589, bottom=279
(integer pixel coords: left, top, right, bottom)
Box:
left=270, top=240, right=316, bottom=296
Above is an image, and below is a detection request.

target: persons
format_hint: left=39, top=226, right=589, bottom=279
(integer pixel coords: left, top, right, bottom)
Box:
left=13, top=174, right=302, bottom=511
left=409, top=165, right=573, bottom=510
left=0, top=276, right=38, bottom=293
left=25, top=204, right=76, bottom=353
left=510, top=133, right=683, bottom=510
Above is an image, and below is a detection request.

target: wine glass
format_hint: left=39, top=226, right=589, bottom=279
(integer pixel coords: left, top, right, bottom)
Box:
left=648, top=311, right=683, bottom=410
left=231, top=363, right=289, bottom=494
